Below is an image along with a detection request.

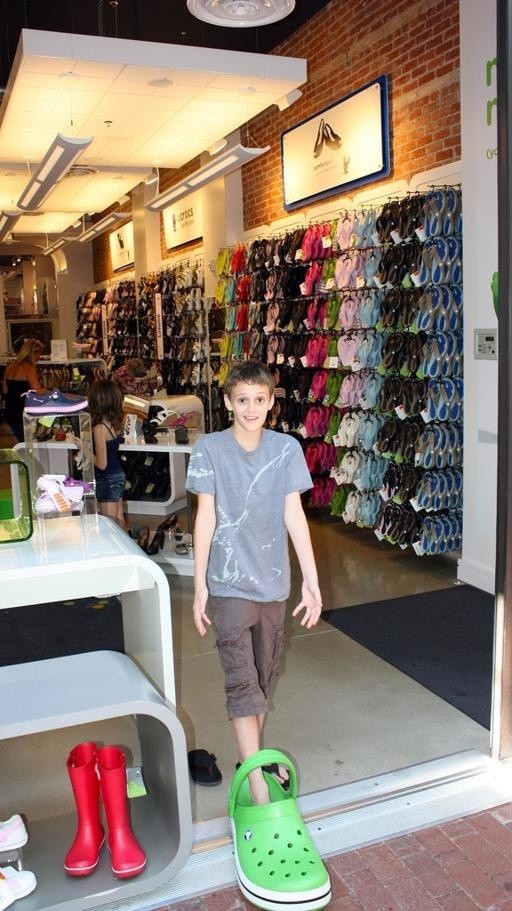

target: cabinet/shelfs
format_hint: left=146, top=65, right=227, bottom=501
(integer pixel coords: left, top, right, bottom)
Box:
left=6, top=433, right=219, bottom=578
left=1, top=449, right=197, bottom=911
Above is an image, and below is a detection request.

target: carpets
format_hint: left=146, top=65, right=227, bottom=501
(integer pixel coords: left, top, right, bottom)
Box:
left=320, top=584, right=493, bottom=732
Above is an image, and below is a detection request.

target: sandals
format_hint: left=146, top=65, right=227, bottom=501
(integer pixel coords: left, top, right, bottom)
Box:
left=142, top=408, right=201, bottom=445
left=314, top=120, right=342, bottom=159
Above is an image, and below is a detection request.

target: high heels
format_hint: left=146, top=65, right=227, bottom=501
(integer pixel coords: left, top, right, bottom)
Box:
left=138, top=512, right=188, bottom=555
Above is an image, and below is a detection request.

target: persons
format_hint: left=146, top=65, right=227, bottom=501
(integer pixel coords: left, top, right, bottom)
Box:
left=74, top=379, right=127, bottom=535
left=3, top=338, right=51, bottom=442
left=184, top=360, right=323, bottom=804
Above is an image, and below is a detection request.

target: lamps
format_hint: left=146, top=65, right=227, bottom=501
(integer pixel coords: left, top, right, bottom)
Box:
left=39, top=208, right=75, bottom=259
left=0, top=173, right=23, bottom=244
left=77, top=175, right=135, bottom=249
left=145, top=84, right=271, bottom=211
left=186, top=0, right=297, bottom=30
left=14, top=69, right=95, bottom=213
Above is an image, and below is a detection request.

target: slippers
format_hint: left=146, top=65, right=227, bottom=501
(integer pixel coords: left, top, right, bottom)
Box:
left=276, top=213, right=378, bottom=373
left=76, top=264, right=209, bottom=385
left=236, top=761, right=292, bottom=790
left=188, top=749, right=223, bottom=785
left=266, top=370, right=464, bottom=471
left=304, top=468, right=463, bottom=556
left=228, top=749, right=334, bottom=911
left=376, top=185, right=461, bottom=375
left=216, top=238, right=279, bottom=387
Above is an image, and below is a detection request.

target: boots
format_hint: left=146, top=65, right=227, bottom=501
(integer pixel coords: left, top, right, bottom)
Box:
left=65, top=744, right=105, bottom=876
left=96, top=746, right=148, bottom=879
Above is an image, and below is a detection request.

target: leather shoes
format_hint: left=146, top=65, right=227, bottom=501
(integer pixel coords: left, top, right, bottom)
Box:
left=35, top=427, right=67, bottom=441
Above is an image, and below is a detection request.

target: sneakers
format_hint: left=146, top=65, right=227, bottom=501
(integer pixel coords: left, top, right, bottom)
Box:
left=20, top=388, right=90, bottom=416
left=1, top=867, right=38, bottom=909
left=35, top=472, right=84, bottom=512
left=1, top=816, right=30, bottom=850
